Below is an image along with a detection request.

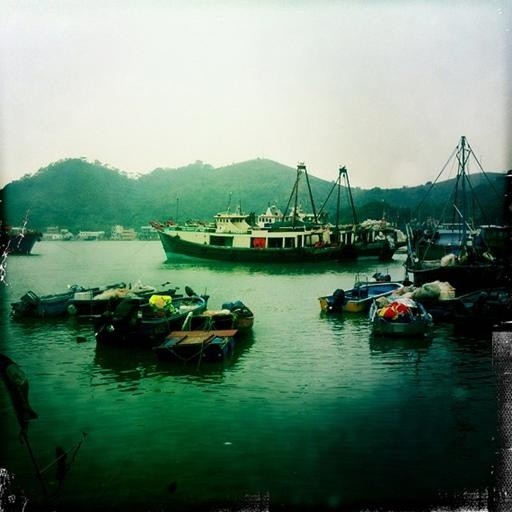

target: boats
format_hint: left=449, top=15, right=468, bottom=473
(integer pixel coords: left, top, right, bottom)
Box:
left=400, top=134, right=511, bottom=313
left=6, top=279, right=256, bottom=371
left=367, top=293, right=434, bottom=337
left=317, top=272, right=404, bottom=315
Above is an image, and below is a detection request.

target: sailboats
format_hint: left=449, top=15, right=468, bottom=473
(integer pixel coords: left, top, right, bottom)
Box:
left=149, top=162, right=408, bottom=265
